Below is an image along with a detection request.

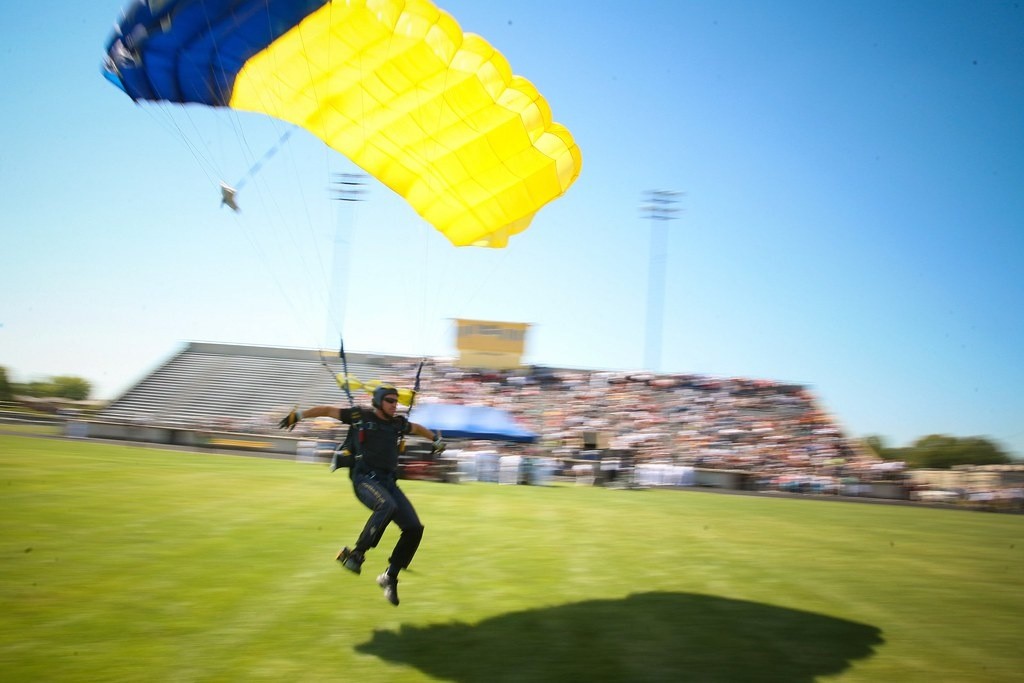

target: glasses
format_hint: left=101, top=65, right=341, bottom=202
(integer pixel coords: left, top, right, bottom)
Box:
left=384, top=398, right=399, bottom=404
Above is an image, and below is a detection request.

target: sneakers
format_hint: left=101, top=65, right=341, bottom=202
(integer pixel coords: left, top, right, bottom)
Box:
left=376, top=573, right=400, bottom=608
left=337, top=547, right=362, bottom=575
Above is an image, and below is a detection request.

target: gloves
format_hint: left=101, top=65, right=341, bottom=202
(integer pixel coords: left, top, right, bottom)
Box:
left=430, top=435, right=446, bottom=455
left=277, top=410, right=301, bottom=432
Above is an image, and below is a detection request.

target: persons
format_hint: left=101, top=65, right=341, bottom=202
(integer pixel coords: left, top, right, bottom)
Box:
left=277, top=386, right=446, bottom=607
left=393, top=353, right=909, bottom=502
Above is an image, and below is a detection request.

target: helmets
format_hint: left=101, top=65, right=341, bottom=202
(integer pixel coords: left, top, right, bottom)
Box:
left=372, top=385, right=399, bottom=410
